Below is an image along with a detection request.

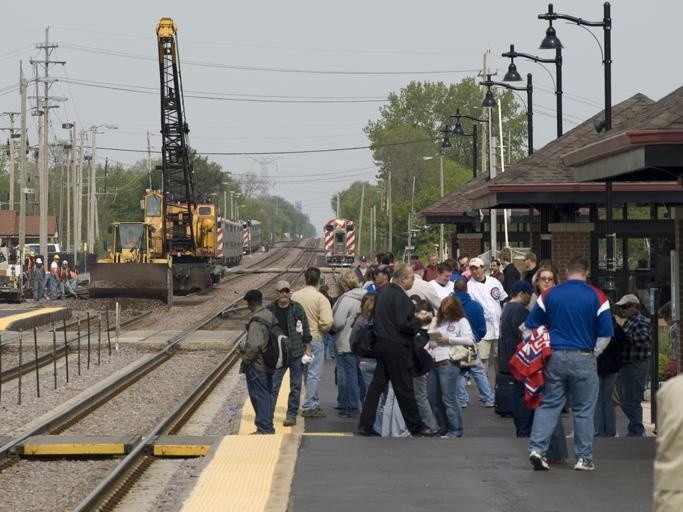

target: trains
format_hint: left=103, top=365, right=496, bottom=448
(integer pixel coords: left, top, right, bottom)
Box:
left=322, top=217, right=355, bottom=268
left=237, top=219, right=262, bottom=254
left=210, top=214, right=244, bottom=269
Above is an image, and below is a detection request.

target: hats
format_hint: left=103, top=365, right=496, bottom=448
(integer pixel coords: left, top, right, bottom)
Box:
left=468, top=258, right=485, bottom=268
left=243, top=290, right=261, bottom=300
left=411, top=260, right=428, bottom=271
left=275, top=280, right=290, bottom=291
left=510, top=281, right=534, bottom=294
left=615, top=294, right=640, bottom=306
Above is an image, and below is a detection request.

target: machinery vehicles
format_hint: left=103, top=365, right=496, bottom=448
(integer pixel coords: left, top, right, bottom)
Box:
left=0, top=253, right=32, bottom=303
left=87, top=219, right=170, bottom=302
left=137, top=17, right=222, bottom=296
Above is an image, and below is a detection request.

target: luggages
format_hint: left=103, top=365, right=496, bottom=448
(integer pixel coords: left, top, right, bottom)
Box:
left=495, top=372, right=517, bottom=418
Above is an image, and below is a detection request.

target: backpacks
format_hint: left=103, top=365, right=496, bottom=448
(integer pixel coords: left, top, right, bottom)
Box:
left=245, top=313, right=290, bottom=369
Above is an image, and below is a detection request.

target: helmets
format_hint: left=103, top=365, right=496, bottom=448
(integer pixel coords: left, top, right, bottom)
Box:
left=29, top=251, right=68, bottom=265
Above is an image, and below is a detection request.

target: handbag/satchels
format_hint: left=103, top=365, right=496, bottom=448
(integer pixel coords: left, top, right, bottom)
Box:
left=350, top=323, right=375, bottom=358
left=459, top=341, right=480, bottom=367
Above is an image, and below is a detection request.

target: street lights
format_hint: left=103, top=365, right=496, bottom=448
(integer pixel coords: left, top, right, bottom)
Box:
left=213, top=178, right=248, bottom=223
left=476, top=69, right=535, bottom=159
left=437, top=122, right=476, bottom=177
left=450, top=106, right=494, bottom=175
left=10, top=110, right=120, bottom=274
left=536, top=1, right=617, bottom=296
left=500, top=40, right=562, bottom=138
left=423, top=152, right=446, bottom=263
left=496, top=131, right=512, bottom=166
left=373, top=158, right=393, bottom=253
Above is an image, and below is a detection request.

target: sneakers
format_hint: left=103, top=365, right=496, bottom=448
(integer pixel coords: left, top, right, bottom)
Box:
left=283, top=415, right=296, bottom=425
left=529, top=450, right=550, bottom=471
left=302, top=406, right=326, bottom=418
left=574, top=458, right=595, bottom=471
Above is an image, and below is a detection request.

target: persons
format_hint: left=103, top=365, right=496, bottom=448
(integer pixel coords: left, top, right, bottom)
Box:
left=239, top=246, right=653, bottom=473
left=655, top=301, right=683, bottom=511
left=20, top=251, right=80, bottom=301
